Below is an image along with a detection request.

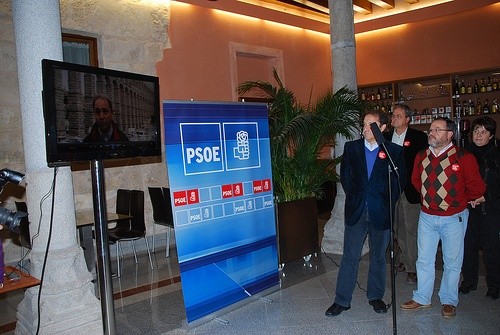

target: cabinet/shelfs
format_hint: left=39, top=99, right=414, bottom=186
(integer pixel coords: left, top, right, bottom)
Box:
left=357, top=65, right=500, bottom=149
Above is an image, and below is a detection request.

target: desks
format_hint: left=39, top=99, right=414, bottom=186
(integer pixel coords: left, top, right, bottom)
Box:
left=0, top=265, right=41, bottom=295
left=75, top=210, right=133, bottom=283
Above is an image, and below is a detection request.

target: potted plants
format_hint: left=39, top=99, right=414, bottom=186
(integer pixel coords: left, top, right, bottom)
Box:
left=232, top=65, right=385, bottom=265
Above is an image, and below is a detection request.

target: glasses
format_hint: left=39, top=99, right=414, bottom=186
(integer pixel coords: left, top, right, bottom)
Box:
left=427, top=129, right=452, bottom=135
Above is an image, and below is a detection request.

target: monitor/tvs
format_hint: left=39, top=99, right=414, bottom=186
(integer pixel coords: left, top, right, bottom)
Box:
left=42, top=58, right=161, bottom=161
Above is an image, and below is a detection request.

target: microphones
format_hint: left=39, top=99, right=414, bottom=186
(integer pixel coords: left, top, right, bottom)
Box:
left=370, top=121, right=400, bottom=177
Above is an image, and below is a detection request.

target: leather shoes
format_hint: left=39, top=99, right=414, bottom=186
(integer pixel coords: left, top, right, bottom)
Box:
left=325, top=302, right=350, bottom=317
left=369, top=299, right=387, bottom=313
left=400, top=299, right=432, bottom=311
left=442, top=304, right=456, bottom=317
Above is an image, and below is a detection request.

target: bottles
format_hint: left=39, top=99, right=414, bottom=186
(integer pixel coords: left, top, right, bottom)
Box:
left=453, top=76, right=500, bottom=149
left=358, top=85, right=394, bottom=127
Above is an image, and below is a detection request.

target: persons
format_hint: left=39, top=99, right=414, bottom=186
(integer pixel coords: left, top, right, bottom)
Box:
left=380, top=103, right=431, bottom=282
left=82, top=96, right=130, bottom=146
left=400, top=117, right=489, bottom=317
left=324, top=109, right=406, bottom=316
left=460, top=117, right=500, bottom=300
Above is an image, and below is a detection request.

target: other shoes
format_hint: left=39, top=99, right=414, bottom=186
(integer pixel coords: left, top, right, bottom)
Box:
left=396, top=263, right=406, bottom=272
left=485, top=288, right=500, bottom=299
left=458, top=283, right=477, bottom=295
left=407, top=272, right=417, bottom=285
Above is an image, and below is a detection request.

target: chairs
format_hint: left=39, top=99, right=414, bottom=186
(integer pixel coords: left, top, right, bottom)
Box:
left=91, top=186, right=174, bottom=278
